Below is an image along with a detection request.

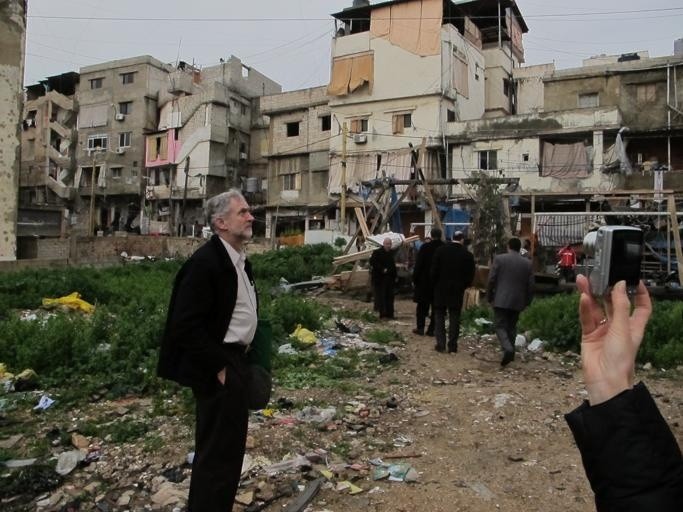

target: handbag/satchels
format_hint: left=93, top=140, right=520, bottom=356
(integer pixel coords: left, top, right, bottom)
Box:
left=248, top=319, right=272, bottom=410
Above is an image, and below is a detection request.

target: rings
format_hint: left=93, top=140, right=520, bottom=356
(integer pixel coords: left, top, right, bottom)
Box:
left=599, top=318, right=607, bottom=324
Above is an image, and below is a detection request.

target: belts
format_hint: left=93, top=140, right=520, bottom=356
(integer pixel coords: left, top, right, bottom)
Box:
left=225, top=343, right=251, bottom=355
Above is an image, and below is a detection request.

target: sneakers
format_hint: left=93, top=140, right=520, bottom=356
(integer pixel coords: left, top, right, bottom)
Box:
left=380, top=315, right=394, bottom=320
left=425, top=331, right=434, bottom=336
left=412, top=329, right=424, bottom=336
left=500, top=350, right=515, bottom=366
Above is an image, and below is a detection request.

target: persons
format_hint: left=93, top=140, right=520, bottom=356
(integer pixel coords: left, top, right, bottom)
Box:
left=431, top=230, right=475, bottom=353
left=156, top=187, right=259, bottom=512
left=564, top=273, right=683, bottom=512
left=369, top=237, right=398, bottom=320
left=487, top=238, right=536, bottom=366
left=557, top=243, right=577, bottom=283
left=412, top=227, right=446, bottom=336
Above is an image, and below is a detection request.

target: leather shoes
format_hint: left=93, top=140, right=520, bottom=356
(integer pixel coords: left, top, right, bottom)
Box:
left=434, top=344, right=457, bottom=354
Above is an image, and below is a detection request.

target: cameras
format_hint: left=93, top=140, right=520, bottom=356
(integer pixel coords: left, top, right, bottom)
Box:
left=583, top=226, right=643, bottom=297
